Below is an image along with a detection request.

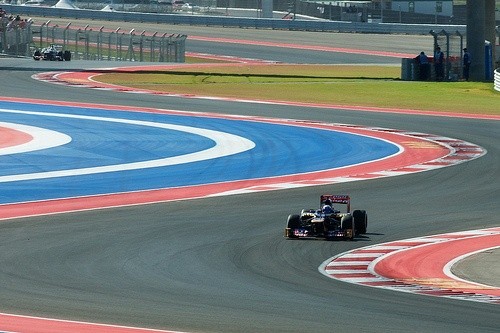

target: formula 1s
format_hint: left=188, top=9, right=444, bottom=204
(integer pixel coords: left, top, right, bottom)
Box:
left=281, top=190, right=368, bottom=241
left=32, top=41, right=72, bottom=62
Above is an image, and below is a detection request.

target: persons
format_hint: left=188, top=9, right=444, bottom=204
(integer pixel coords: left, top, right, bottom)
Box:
left=413, top=45, right=470, bottom=82
